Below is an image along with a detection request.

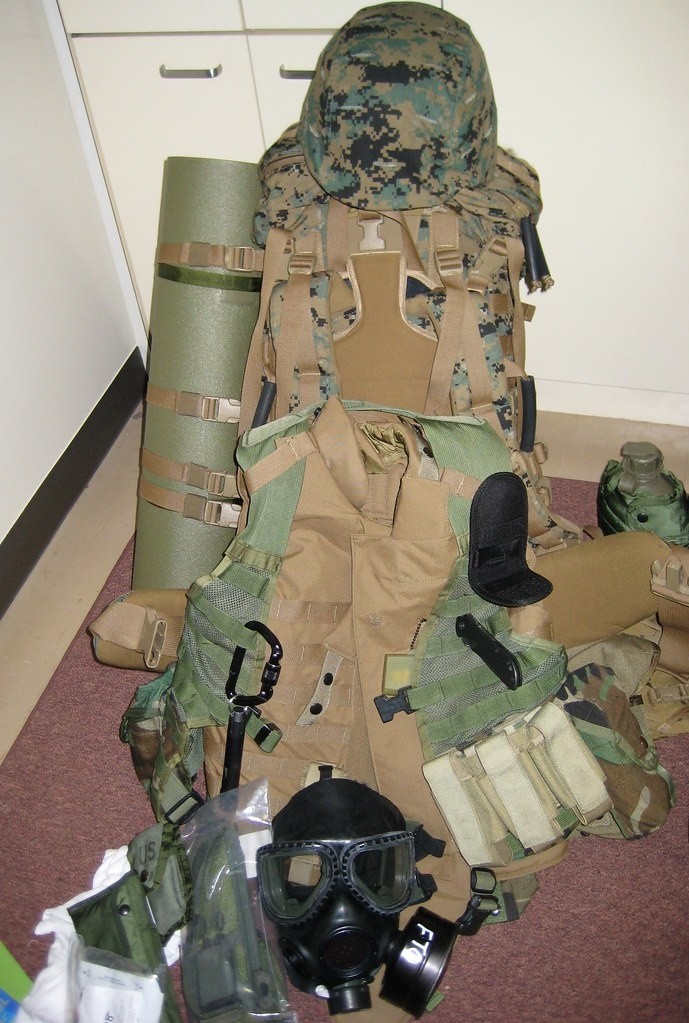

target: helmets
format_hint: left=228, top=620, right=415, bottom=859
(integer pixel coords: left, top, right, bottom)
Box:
left=297, top=1, right=497, bottom=211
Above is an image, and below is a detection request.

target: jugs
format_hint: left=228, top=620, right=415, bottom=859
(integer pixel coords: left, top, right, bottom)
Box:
left=596, top=442, right=689, bottom=549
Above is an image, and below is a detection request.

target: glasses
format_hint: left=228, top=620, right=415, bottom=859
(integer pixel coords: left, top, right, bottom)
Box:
left=256, top=829, right=418, bottom=927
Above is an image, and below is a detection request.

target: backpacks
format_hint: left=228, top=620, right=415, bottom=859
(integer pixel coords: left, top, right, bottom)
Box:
left=236, top=145, right=553, bottom=540
